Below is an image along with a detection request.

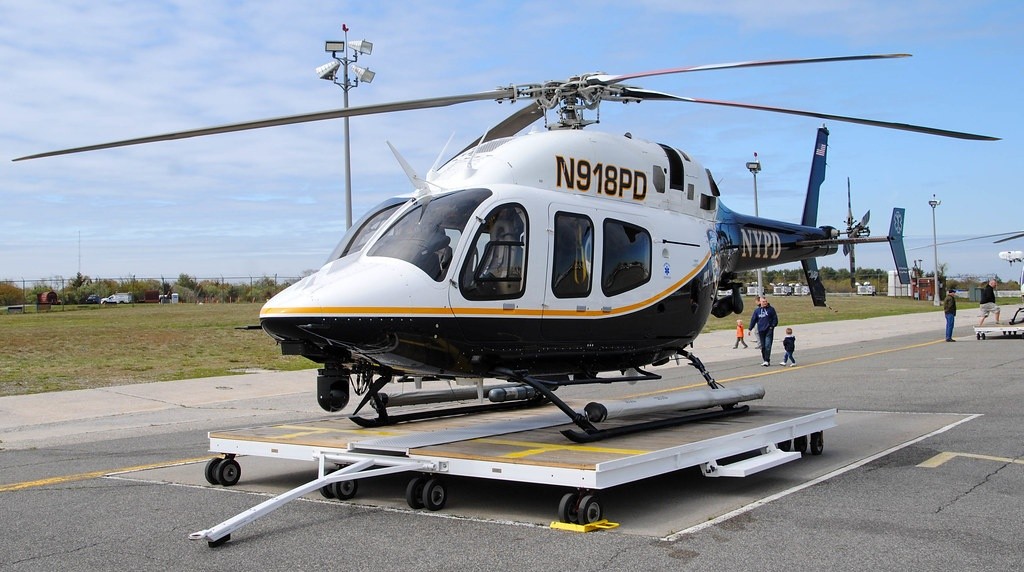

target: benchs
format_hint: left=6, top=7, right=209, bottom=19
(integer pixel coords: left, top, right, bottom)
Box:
left=8, top=307, right=22, bottom=313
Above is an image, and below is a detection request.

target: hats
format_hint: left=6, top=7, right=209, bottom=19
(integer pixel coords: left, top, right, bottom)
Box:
left=949, top=289, right=956, bottom=293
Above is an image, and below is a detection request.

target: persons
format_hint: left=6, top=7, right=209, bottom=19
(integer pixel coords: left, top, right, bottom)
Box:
left=733, top=295, right=797, bottom=367
left=977, top=279, right=1003, bottom=326
left=944, top=289, right=956, bottom=342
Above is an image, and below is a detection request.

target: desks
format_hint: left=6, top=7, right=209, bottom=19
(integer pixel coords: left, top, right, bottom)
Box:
left=35, top=302, right=51, bottom=313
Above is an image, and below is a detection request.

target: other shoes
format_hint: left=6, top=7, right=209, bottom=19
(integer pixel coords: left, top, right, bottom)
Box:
left=946, top=339, right=955, bottom=342
left=743, top=345, right=748, bottom=348
left=733, top=347, right=737, bottom=349
left=790, top=363, right=797, bottom=367
left=761, top=361, right=770, bottom=366
left=780, top=362, right=786, bottom=365
left=996, top=321, right=1002, bottom=325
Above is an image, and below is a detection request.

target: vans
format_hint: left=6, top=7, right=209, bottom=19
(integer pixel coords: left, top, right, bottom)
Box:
left=100, top=292, right=132, bottom=305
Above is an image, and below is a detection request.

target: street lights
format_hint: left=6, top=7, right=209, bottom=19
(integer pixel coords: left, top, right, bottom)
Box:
left=744, top=150, right=765, bottom=299
left=926, top=192, right=944, bottom=306
left=315, top=22, right=377, bottom=233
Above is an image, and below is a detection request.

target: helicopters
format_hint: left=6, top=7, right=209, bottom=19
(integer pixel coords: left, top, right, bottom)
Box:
left=904, top=229, right=1024, bottom=326
left=8, top=52, right=1005, bottom=443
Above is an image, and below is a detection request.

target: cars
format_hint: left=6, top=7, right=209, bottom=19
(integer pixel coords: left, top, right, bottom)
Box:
left=85, top=295, right=101, bottom=304
